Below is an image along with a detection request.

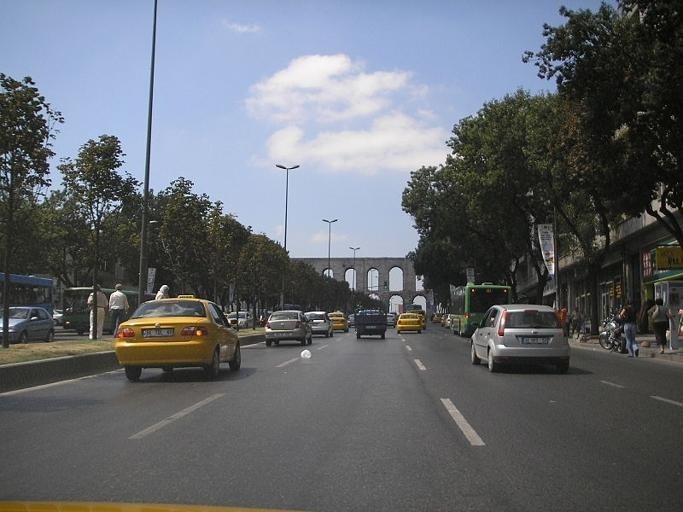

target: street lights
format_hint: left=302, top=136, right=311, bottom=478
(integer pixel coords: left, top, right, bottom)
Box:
left=349, top=247, right=362, bottom=290
left=320, top=218, right=339, bottom=280
left=275, top=164, right=302, bottom=310
left=137, top=220, right=161, bottom=308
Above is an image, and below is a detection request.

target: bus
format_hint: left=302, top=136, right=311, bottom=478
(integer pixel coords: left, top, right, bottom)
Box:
left=0, top=272, right=55, bottom=325
left=448, top=280, right=513, bottom=338
left=62, top=286, right=158, bottom=336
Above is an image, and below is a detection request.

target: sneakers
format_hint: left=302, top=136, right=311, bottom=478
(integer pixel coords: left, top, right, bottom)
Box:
left=628, top=349, right=639, bottom=358
left=660, top=349, right=665, bottom=354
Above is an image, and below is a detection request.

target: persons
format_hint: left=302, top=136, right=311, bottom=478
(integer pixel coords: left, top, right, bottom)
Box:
left=108, top=283, right=128, bottom=338
left=571, top=307, right=581, bottom=334
left=154, top=285, right=169, bottom=299
left=619, top=301, right=639, bottom=357
left=648, top=298, right=670, bottom=353
left=88, top=284, right=109, bottom=339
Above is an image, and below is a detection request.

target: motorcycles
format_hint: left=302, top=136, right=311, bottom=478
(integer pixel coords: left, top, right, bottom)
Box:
left=598, top=318, right=626, bottom=353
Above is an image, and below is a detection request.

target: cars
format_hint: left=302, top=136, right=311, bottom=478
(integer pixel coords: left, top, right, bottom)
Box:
left=56, top=309, right=63, bottom=314
left=221, top=302, right=453, bottom=339
left=0, top=304, right=55, bottom=346
left=110, top=293, right=243, bottom=383
left=53, top=309, right=64, bottom=326
left=262, top=308, right=312, bottom=347
left=467, top=302, right=572, bottom=375
